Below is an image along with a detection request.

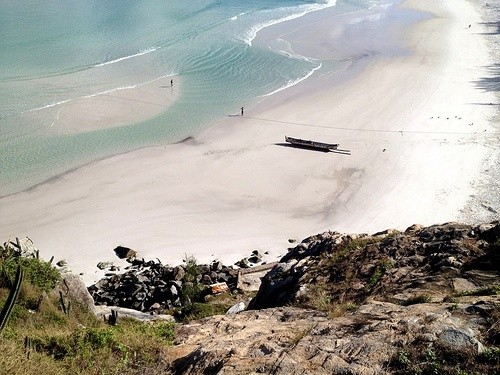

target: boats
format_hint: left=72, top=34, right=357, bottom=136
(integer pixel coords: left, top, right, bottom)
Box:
left=284, top=134, right=351, bottom=156
